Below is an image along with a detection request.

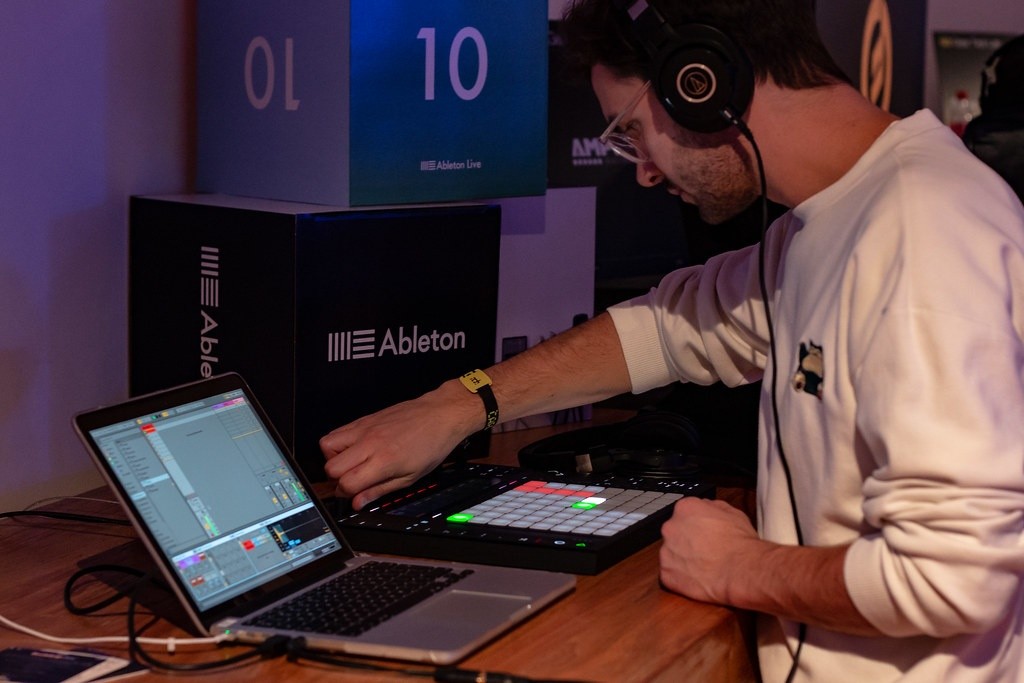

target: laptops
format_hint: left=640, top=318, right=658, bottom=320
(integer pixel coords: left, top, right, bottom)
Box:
left=72, top=371, right=576, bottom=664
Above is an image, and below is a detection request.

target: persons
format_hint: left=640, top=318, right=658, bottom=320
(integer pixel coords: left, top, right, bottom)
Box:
left=320, top=0, right=1023, bottom=683
left=961, top=33, right=1024, bottom=203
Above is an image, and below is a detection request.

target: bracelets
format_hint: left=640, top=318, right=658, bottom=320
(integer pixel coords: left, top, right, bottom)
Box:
left=458, top=368, right=498, bottom=435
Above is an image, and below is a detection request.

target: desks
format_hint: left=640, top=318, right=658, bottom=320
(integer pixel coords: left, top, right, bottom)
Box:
left=0, top=410, right=761, bottom=683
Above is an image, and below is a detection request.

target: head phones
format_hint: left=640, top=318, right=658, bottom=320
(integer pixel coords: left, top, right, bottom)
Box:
left=626, top=0, right=755, bottom=134
left=518, top=412, right=716, bottom=500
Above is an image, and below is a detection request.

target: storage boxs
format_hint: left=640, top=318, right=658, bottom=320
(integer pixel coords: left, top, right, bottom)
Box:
left=197, top=0, right=549, bottom=209
left=129, top=186, right=596, bottom=484
